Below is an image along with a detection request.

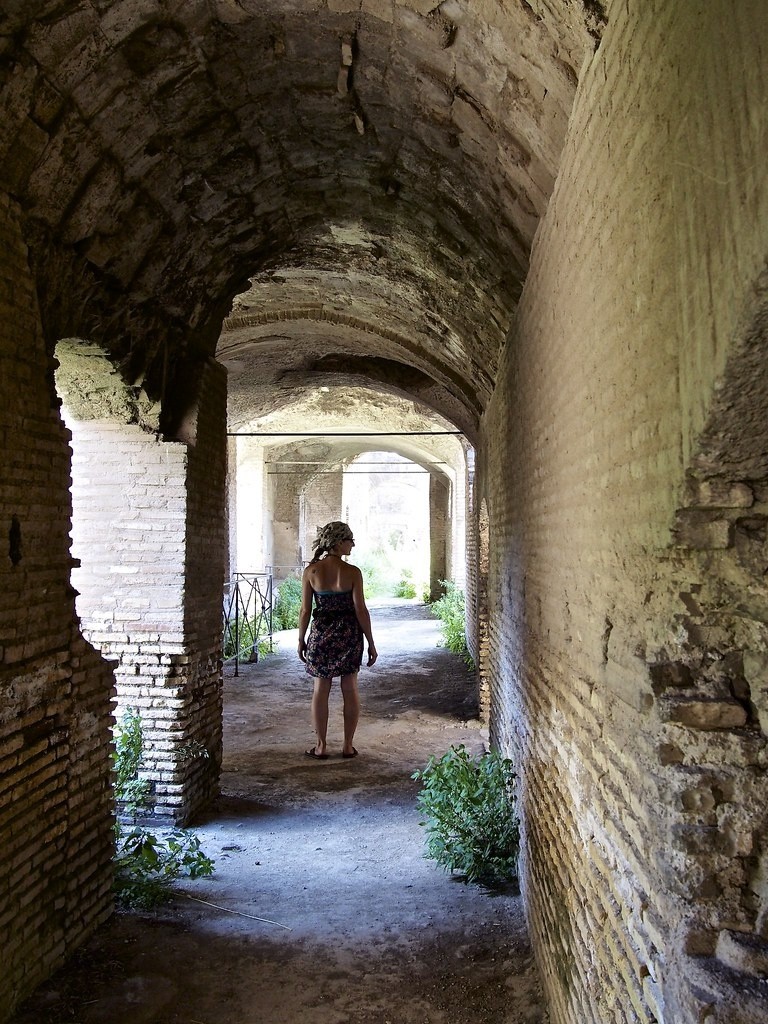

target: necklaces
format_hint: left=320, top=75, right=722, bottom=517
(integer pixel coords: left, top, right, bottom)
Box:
left=325, top=554, right=340, bottom=558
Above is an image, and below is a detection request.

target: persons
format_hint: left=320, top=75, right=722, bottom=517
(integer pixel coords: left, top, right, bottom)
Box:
left=297, top=521, right=378, bottom=760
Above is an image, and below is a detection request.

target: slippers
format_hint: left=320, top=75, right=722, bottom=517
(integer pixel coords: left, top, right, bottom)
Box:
left=342, top=746, right=358, bottom=758
left=304, top=747, right=328, bottom=759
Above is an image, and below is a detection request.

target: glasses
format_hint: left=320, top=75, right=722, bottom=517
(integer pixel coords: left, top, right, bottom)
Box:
left=343, top=538, right=355, bottom=542
